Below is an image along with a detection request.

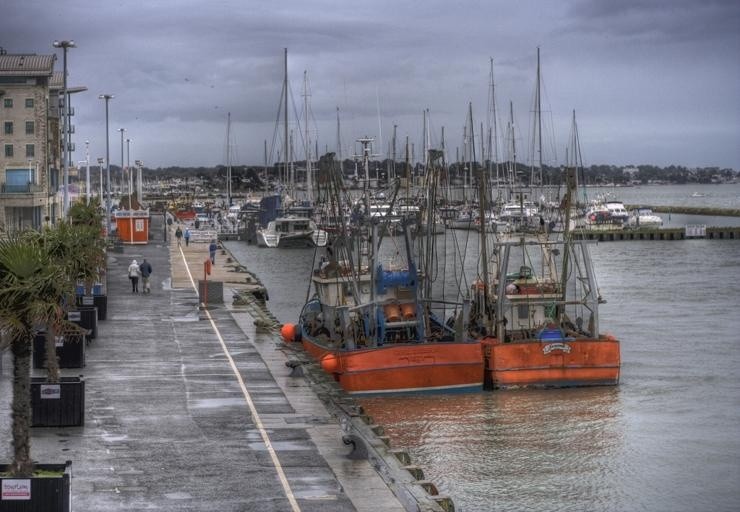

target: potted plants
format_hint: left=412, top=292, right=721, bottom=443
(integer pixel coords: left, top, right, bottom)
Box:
left=0, top=195, right=110, bottom=511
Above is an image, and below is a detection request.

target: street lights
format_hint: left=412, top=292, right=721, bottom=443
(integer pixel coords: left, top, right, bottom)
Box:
left=135, top=157, right=145, bottom=207
left=117, top=128, right=128, bottom=199
left=98, top=157, right=104, bottom=217
left=53, top=38, right=77, bottom=230
left=97, top=93, right=116, bottom=235
left=123, top=138, right=133, bottom=194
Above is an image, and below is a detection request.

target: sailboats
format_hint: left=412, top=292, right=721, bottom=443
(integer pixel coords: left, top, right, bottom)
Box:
left=165, top=48, right=664, bottom=249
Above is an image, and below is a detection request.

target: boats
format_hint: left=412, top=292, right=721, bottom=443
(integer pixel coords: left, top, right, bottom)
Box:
left=454, top=173, right=620, bottom=390
left=281, top=138, right=485, bottom=398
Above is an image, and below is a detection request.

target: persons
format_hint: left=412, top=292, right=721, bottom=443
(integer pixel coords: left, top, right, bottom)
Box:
left=168, top=215, right=190, bottom=247
left=128, top=259, right=142, bottom=292
left=210, top=239, right=217, bottom=264
left=195, top=218, right=200, bottom=229
left=140, top=259, right=152, bottom=292
